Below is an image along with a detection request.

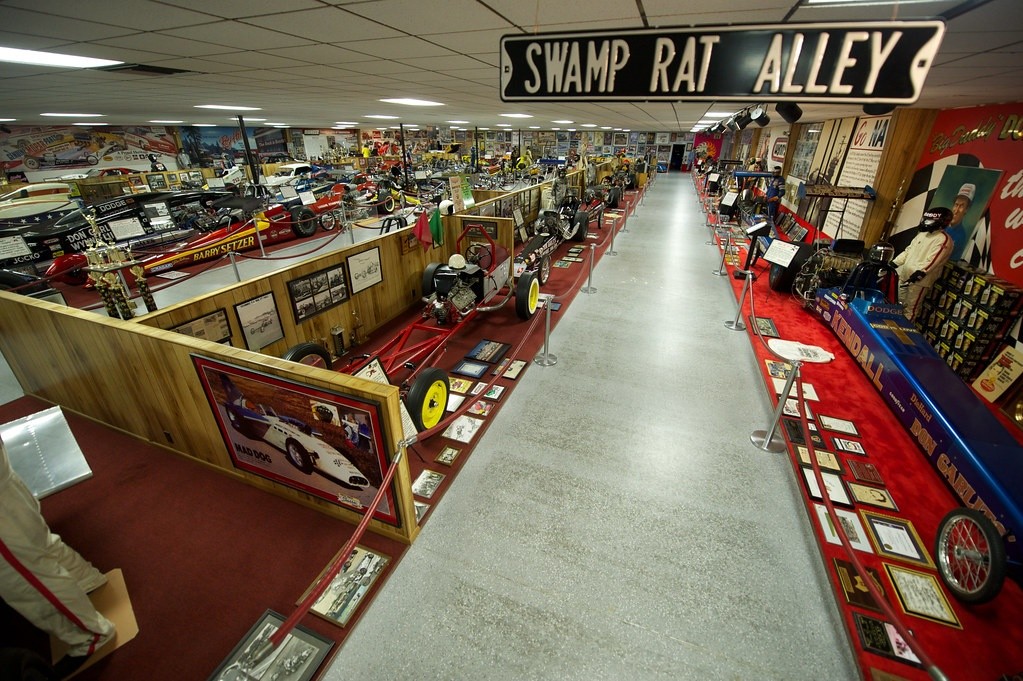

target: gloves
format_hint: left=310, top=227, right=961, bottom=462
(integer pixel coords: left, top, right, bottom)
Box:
left=877, top=261, right=898, bottom=277
left=900, top=270, right=926, bottom=288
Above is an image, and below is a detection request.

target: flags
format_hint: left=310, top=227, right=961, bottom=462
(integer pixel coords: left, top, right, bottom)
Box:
left=429, top=209, right=443, bottom=244
left=411, top=210, right=432, bottom=251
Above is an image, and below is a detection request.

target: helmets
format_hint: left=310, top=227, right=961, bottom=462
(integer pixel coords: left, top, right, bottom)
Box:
left=917, top=206, right=953, bottom=232
left=750, top=157, right=755, bottom=163
left=439, top=199, right=455, bottom=216
left=448, top=253, right=466, bottom=270
left=557, top=166, right=567, bottom=178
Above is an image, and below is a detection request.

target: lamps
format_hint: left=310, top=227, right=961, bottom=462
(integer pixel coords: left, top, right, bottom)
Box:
left=775, top=102, right=803, bottom=124
left=726, top=115, right=738, bottom=132
left=711, top=122, right=720, bottom=133
left=717, top=121, right=727, bottom=133
left=705, top=125, right=712, bottom=135
left=751, top=103, right=770, bottom=127
left=304, top=130, right=320, bottom=135
left=736, top=107, right=753, bottom=130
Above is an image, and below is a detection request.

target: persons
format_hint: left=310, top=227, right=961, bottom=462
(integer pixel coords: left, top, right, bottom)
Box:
left=525, top=146, right=532, bottom=170
left=124, top=244, right=135, bottom=262
left=81, top=249, right=91, bottom=267
left=511, top=146, right=518, bottom=173
left=889, top=207, right=953, bottom=322
left=763, top=166, right=785, bottom=220
left=552, top=169, right=570, bottom=208
left=295, top=172, right=311, bottom=192
left=743, top=157, right=762, bottom=190
left=98, top=251, right=107, bottom=268
left=80, top=209, right=102, bottom=240
left=148, top=154, right=168, bottom=172
left=0, top=436, right=118, bottom=681
left=686, top=147, right=698, bottom=171
left=362, top=144, right=371, bottom=157
left=177, top=148, right=193, bottom=169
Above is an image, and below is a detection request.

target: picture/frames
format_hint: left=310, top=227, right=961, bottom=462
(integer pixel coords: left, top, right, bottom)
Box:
left=178, top=172, right=190, bottom=182
left=652, top=132, right=963, bottom=672
left=463, top=338, right=512, bottom=365
left=166, top=174, right=178, bottom=182
left=359, top=131, right=652, bottom=168
left=345, top=246, right=385, bottom=296
left=146, top=173, right=168, bottom=191
left=536, top=300, right=563, bottom=312
left=286, top=262, right=351, bottom=325
left=433, top=444, right=463, bottom=467
left=164, top=307, right=233, bottom=343
left=206, top=609, right=336, bottom=681
left=566, top=247, right=584, bottom=254
left=128, top=176, right=144, bottom=186
left=460, top=186, right=540, bottom=245
left=232, top=290, right=285, bottom=352
left=214, top=168, right=224, bottom=178
left=490, top=356, right=528, bottom=381
left=448, top=359, right=490, bottom=379
left=466, top=379, right=510, bottom=403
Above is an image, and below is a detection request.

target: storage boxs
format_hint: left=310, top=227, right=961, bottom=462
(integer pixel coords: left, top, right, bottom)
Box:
left=972, top=340, right=1023, bottom=404
left=912, top=262, right=1023, bottom=384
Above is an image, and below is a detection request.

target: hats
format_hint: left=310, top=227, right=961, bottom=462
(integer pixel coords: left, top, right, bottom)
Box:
left=956, top=184, right=976, bottom=203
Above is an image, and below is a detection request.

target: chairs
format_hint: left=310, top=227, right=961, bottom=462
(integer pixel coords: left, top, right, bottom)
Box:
left=556, top=195, right=576, bottom=224
left=847, top=266, right=892, bottom=305
left=820, top=239, right=864, bottom=273
left=479, top=244, right=508, bottom=271
